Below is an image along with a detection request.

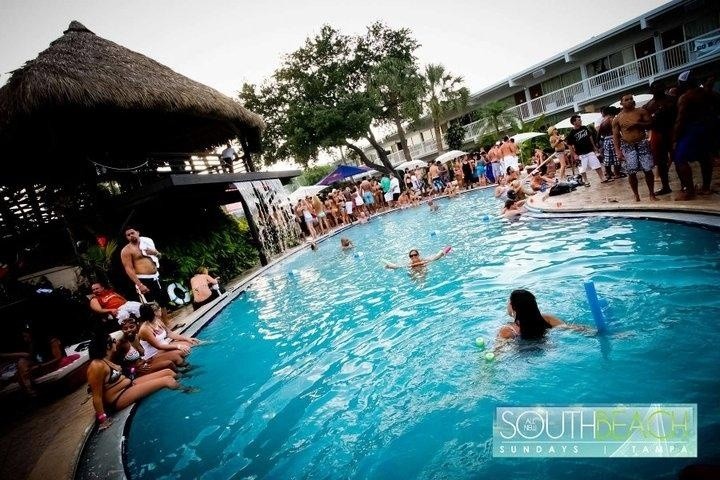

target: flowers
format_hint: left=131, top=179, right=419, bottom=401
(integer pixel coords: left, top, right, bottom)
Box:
left=80, top=231, right=119, bottom=270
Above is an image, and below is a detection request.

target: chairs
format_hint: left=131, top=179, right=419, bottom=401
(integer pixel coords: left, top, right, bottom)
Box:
left=0, top=293, right=160, bottom=407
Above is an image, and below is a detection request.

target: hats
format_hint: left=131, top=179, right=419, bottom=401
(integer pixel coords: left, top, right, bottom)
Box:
left=496, top=141, right=500, bottom=145
left=437, top=161, right=441, bottom=164
left=328, top=189, right=336, bottom=196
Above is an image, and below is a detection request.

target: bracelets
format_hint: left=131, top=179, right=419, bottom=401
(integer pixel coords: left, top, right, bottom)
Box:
left=130, top=367, right=135, bottom=374
left=178, top=344, right=181, bottom=350
left=98, top=413, right=106, bottom=420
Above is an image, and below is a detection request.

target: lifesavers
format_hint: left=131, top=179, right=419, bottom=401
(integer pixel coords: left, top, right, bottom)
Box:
left=168, top=283, right=190, bottom=305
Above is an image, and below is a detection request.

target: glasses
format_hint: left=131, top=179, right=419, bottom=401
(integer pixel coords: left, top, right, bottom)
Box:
left=410, top=254, right=417, bottom=257
left=112, top=338, right=116, bottom=343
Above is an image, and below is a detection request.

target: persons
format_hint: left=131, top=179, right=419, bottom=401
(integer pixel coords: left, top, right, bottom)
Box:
left=7, top=325, right=67, bottom=386
left=84, top=332, right=201, bottom=432
left=189, top=264, right=224, bottom=310
left=87, top=282, right=125, bottom=314
left=222, top=143, right=238, bottom=173
left=378, top=246, right=453, bottom=269
left=118, top=223, right=172, bottom=324
left=110, top=318, right=206, bottom=379
left=491, top=285, right=565, bottom=346
left=135, top=301, right=224, bottom=357
left=274, top=64, right=719, bottom=254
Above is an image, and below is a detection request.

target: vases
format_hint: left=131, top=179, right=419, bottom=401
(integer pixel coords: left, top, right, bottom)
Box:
left=102, top=268, right=115, bottom=292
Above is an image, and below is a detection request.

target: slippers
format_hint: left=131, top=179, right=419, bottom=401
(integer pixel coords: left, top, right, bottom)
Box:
left=654, top=188, right=672, bottom=196
left=605, top=171, right=628, bottom=180
left=600, top=179, right=612, bottom=184
left=584, top=183, right=590, bottom=187
